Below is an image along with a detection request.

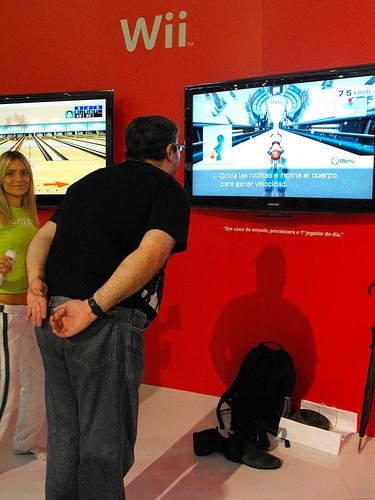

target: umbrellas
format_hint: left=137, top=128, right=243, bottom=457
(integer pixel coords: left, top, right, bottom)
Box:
left=357, top=282, right=375, bottom=453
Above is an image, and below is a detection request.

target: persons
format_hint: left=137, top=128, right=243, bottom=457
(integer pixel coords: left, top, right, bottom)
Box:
left=0, top=151, right=48, bottom=460
left=26, top=117, right=191, bottom=500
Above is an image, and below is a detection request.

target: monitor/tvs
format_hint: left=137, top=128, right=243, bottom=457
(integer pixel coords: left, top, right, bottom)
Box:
left=0, top=89, right=113, bottom=208
left=183, top=63, right=375, bottom=214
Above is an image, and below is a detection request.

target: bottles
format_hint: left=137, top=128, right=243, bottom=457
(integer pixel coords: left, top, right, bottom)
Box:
left=0, top=249, right=18, bottom=287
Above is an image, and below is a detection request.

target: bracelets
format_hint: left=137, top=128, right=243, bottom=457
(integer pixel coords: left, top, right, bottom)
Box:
left=87, top=297, right=106, bottom=318
